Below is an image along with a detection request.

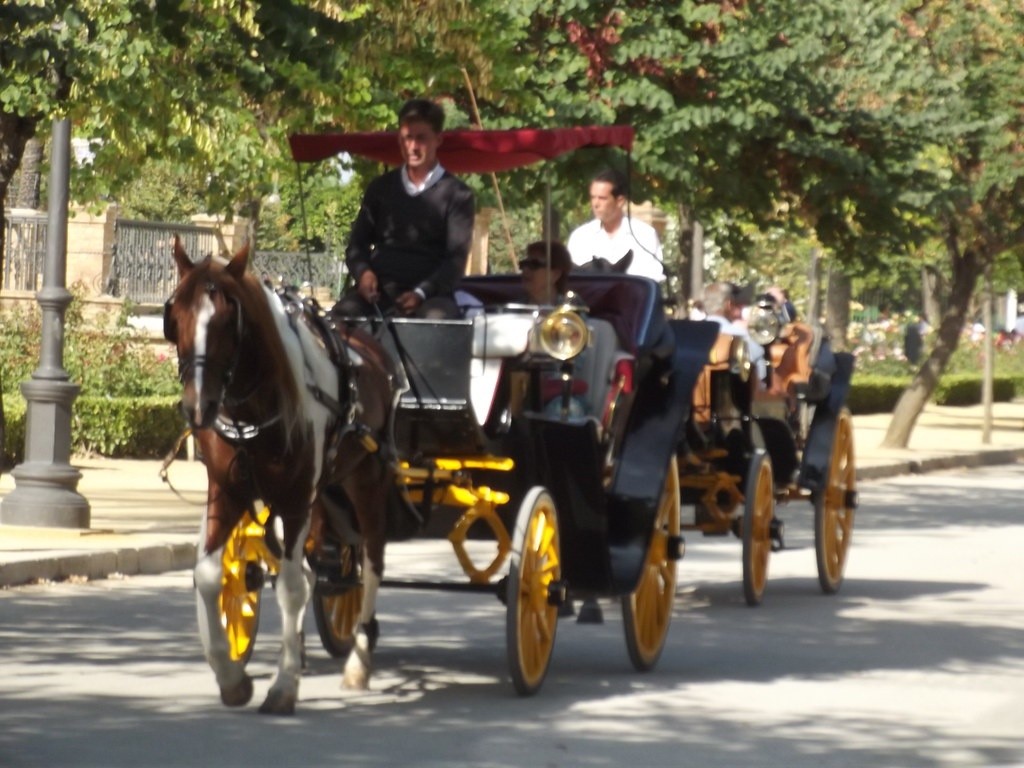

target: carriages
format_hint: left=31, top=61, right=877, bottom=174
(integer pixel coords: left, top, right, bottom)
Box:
left=165, top=125, right=858, bottom=714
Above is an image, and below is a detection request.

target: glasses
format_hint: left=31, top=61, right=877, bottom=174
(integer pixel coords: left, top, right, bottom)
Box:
left=518, top=258, right=551, bottom=271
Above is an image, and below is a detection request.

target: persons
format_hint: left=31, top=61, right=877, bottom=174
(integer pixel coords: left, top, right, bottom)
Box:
left=506, top=238, right=582, bottom=321
left=916, top=313, right=932, bottom=334
left=688, top=281, right=797, bottom=387
left=566, top=169, right=667, bottom=283
left=331, top=98, right=475, bottom=318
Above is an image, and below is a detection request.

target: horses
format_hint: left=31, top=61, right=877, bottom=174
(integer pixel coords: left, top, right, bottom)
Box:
left=556, top=248, right=645, bottom=624
left=162, top=231, right=398, bottom=718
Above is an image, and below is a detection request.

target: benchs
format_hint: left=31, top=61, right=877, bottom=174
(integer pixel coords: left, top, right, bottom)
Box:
left=452, top=301, right=634, bottom=487
left=689, top=318, right=815, bottom=470
